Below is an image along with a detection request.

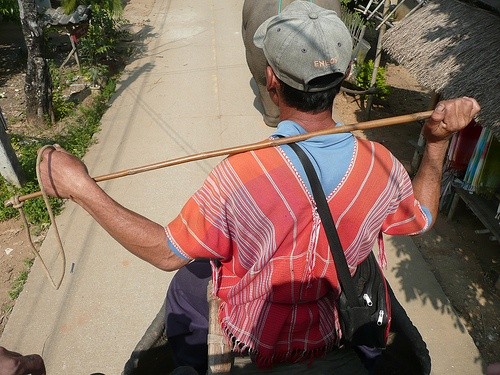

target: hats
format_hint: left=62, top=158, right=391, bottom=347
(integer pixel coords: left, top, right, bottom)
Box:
left=252, top=0, right=354, bottom=93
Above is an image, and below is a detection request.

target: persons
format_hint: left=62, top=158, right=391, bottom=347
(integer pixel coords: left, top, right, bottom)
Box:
left=0, top=346, right=46, bottom=375
left=37, top=0, right=481, bottom=375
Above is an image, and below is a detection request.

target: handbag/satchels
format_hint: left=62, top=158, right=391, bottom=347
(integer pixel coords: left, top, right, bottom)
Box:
left=337, top=250, right=392, bottom=349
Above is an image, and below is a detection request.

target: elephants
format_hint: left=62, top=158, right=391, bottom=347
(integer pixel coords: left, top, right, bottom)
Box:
left=242, top=0, right=341, bottom=126
